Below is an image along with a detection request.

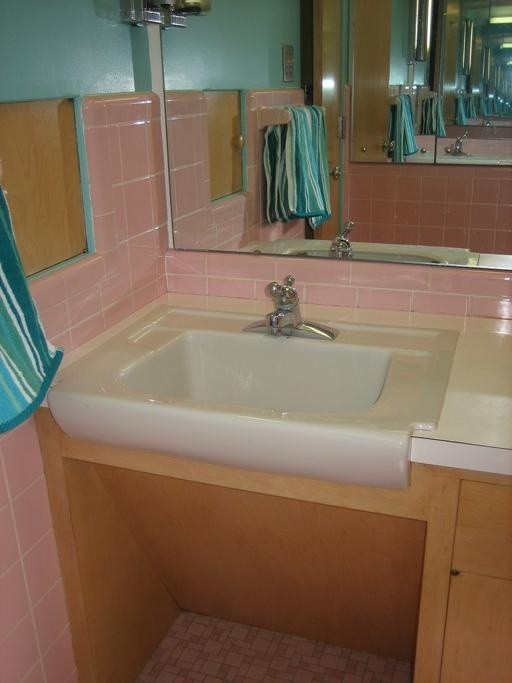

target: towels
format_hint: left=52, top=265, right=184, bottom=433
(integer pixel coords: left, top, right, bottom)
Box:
left=0, top=189, right=64, bottom=437
left=264, top=103, right=333, bottom=231
left=454, top=89, right=508, bottom=125
left=386, top=94, right=418, bottom=164
left=422, top=96, right=446, bottom=137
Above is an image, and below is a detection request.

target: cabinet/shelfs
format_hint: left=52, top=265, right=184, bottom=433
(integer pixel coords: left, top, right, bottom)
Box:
left=412, top=469, right=511, bottom=682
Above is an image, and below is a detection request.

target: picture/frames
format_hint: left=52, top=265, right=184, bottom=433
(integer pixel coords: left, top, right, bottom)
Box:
left=283, top=45, right=293, bottom=82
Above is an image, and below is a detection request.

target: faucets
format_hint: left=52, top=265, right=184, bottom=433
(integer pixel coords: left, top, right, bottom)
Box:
left=485, top=117, right=492, bottom=127
left=266, top=274, right=303, bottom=337
left=329, top=219, right=356, bottom=252
left=445, top=130, right=472, bottom=156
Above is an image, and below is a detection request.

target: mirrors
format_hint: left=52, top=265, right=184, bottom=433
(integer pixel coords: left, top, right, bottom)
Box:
left=442, top=1, right=512, bottom=128
left=347, top=1, right=436, bottom=165
left=434, top=0, right=512, bottom=166
left=158, top=0, right=512, bottom=271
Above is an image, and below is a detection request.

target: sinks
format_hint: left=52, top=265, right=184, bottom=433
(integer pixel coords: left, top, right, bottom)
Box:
left=281, top=247, right=450, bottom=266
left=117, top=330, right=393, bottom=416
